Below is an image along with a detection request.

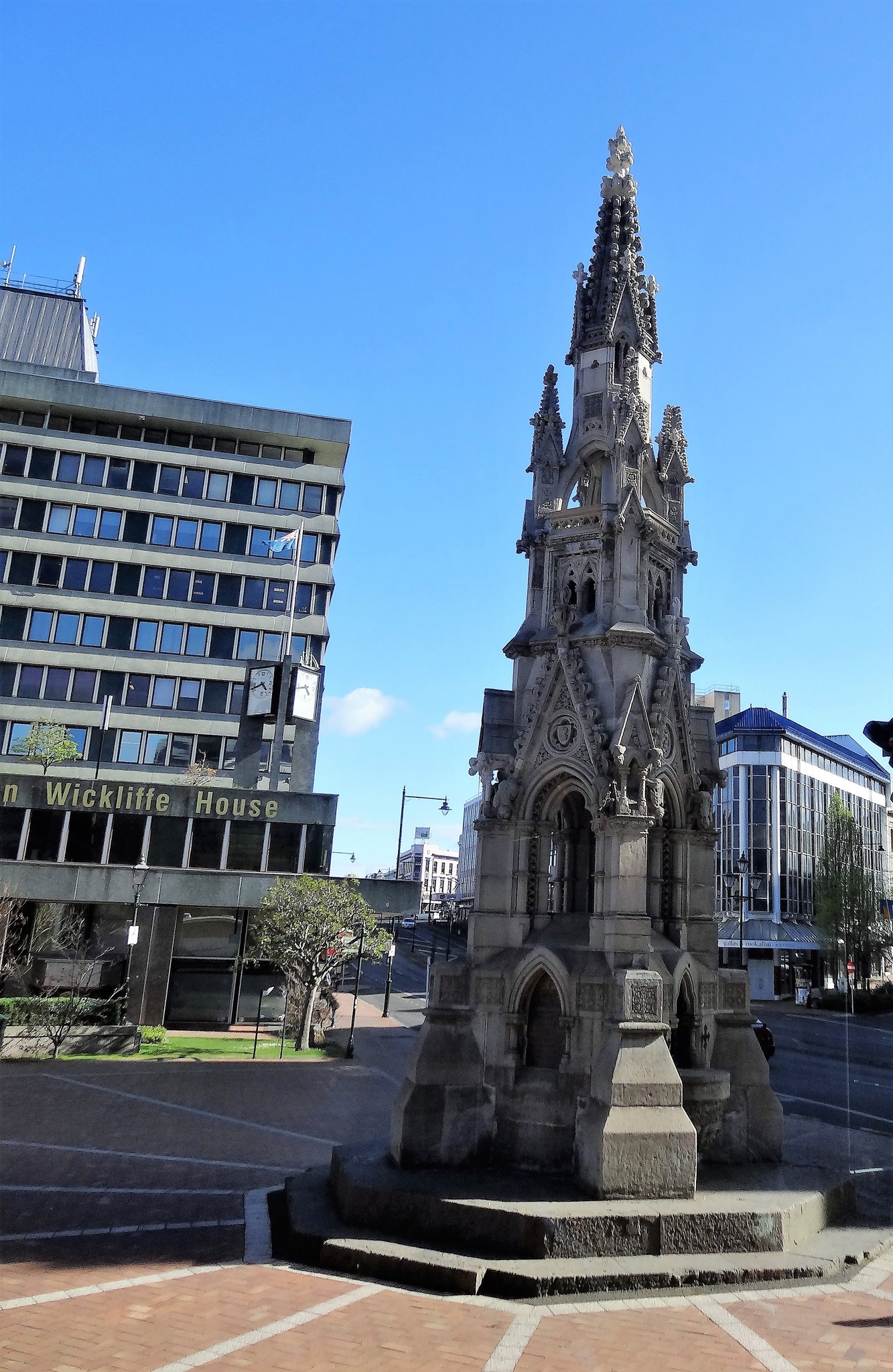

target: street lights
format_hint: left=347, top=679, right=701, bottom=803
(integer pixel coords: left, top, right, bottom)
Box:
left=346, top=918, right=366, bottom=1062
left=125, top=854, right=150, bottom=1028
left=446, top=895, right=454, bottom=962
left=724, top=854, right=763, bottom=970
left=396, top=784, right=451, bottom=882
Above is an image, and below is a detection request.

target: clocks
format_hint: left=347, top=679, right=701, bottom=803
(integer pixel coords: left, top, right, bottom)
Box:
left=242, top=662, right=279, bottom=718
left=287, top=661, right=326, bottom=724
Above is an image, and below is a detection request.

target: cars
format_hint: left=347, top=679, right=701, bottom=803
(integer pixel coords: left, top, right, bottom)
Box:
left=402, top=917, right=417, bottom=929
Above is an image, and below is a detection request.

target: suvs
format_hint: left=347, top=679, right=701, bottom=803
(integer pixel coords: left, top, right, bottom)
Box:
left=420, top=910, right=440, bottom=920
